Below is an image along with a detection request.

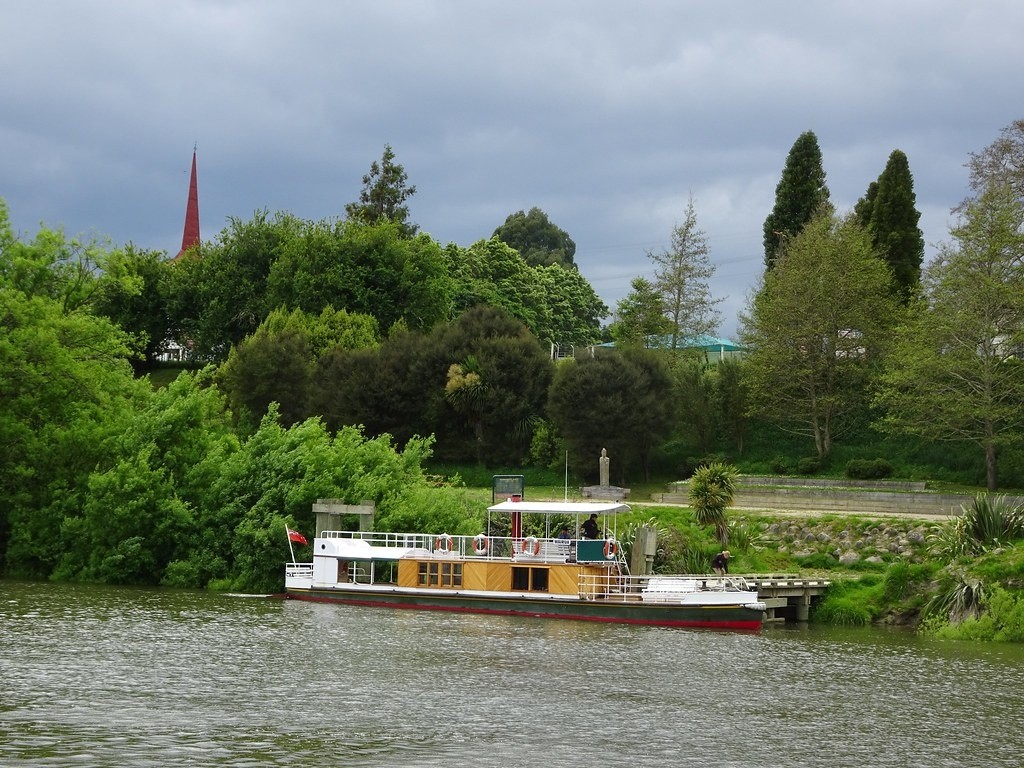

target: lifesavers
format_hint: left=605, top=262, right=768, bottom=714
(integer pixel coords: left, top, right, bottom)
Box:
left=434, top=532, right=455, bottom=556
left=520, top=535, right=539, bottom=559
left=472, top=532, right=490, bottom=556
left=603, top=538, right=618, bottom=560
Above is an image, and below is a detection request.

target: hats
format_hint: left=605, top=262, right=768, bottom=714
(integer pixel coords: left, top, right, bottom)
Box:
left=590, top=513, right=598, bottom=518
left=724, top=551, right=735, bottom=558
left=562, top=526, right=570, bottom=531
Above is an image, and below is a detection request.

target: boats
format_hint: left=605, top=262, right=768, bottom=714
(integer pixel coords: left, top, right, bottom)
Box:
left=282, top=495, right=767, bottom=634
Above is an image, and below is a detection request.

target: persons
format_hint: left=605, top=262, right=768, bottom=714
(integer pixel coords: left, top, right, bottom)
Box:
left=711, top=551, right=730, bottom=588
left=558, top=527, right=570, bottom=539
left=580, top=514, right=600, bottom=540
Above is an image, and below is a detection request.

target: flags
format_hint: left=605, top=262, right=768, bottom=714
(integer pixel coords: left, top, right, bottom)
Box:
left=288, top=528, right=307, bottom=545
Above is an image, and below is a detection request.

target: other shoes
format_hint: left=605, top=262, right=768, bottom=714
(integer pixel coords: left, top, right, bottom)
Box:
left=717, top=582, right=725, bottom=587
